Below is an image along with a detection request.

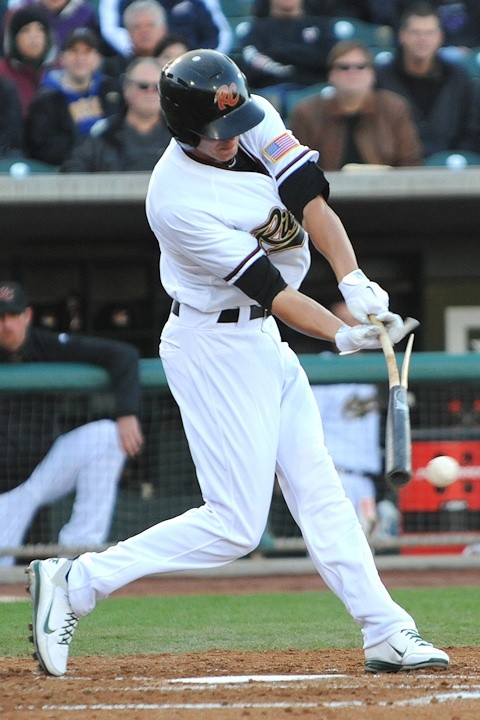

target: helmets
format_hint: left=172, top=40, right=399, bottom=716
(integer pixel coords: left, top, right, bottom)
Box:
left=157, top=48, right=265, bottom=148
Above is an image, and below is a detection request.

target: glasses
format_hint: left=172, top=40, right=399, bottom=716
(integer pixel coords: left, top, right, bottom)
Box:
left=124, top=79, right=158, bottom=91
left=331, top=60, right=368, bottom=73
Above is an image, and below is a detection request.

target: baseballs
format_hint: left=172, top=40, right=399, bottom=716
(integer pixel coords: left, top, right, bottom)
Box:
left=424, top=456, right=461, bottom=489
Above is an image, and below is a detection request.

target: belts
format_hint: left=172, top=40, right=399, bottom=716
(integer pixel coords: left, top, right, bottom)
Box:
left=171, top=300, right=265, bottom=323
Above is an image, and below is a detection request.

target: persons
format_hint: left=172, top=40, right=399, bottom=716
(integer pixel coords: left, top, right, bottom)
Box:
left=25, top=48, right=451, bottom=676
left=0, top=0, right=480, bottom=562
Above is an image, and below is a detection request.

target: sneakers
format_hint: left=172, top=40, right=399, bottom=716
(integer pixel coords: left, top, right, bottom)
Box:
left=24, top=555, right=79, bottom=677
left=364, top=629, right=450, bottom=669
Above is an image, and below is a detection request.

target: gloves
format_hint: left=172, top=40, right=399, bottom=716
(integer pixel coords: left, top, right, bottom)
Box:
left=337, top=269, right=389, bottom=328
left=335, top=310, right=420, bottom=355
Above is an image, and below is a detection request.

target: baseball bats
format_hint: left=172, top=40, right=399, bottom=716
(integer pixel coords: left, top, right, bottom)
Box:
left=366, top=314, right=417, bottom=488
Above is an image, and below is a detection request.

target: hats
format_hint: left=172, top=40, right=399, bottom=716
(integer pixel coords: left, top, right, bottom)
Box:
left=0, top=279, right=29, bottom=314
left=59, top=28, right=96, bottom=50
